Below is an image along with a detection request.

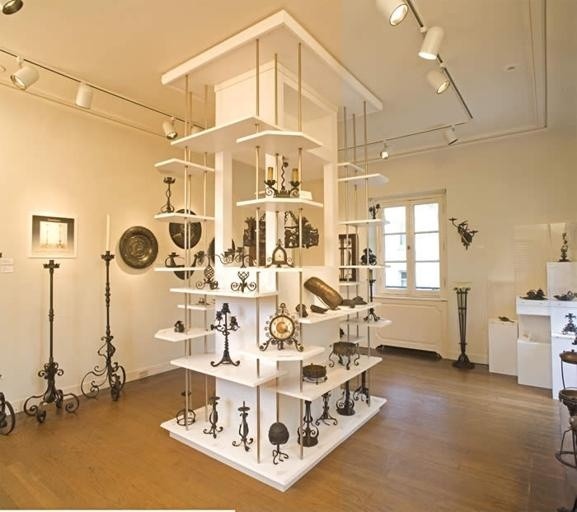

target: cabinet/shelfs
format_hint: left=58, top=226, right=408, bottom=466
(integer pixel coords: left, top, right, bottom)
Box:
left=155, top=8, right=388, bottom=492
left=487, top=259, right=575, bottom=400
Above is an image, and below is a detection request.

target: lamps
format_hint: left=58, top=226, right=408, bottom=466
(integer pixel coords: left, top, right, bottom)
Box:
left=9, top=56, right=93, bottom=110
left=162, top=116, right=178, bottom=140
left=374, top=1, right=450, bottom=94
left=444, top=124, right=457, bottom=147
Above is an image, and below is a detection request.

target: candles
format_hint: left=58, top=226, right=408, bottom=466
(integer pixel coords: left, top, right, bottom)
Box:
left=106, top=214, right=110, bottom=251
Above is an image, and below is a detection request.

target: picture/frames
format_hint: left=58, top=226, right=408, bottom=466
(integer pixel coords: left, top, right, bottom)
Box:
left=26, top=208, right=78, bottom=259
